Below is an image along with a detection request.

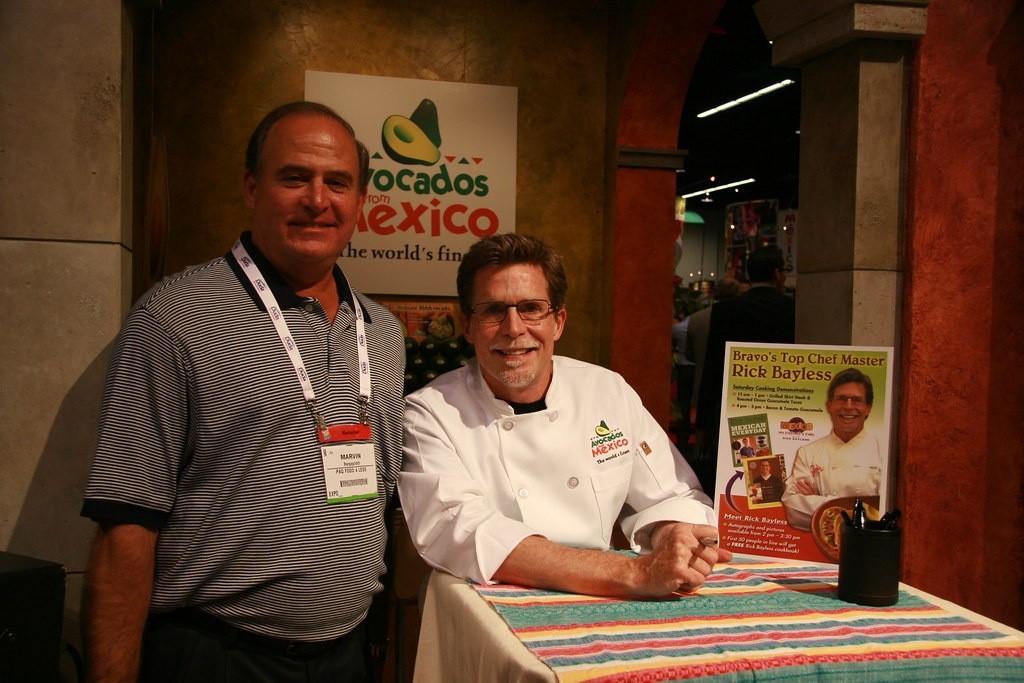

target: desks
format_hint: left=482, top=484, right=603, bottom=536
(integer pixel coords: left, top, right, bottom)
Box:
left=412, top=548, right=1024, bottom=683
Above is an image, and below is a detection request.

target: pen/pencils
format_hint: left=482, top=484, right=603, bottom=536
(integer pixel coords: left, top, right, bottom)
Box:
left=698, top=536, right=718, bottom=547
left=841, top=510, right=853, bottom=526
left=854, top=498, right=863, bottom=528
left=874, top=509, right=902, bottom=530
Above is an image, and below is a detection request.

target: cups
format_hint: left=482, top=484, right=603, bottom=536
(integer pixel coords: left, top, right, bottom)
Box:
left=837, top=520, right=902, bottom=608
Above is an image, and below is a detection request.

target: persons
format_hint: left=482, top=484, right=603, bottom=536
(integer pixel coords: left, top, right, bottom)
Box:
left=740, top=438, right=754, bottom=458
left=394, top=233, right=732, bottom=602
left=754, top=459, right=784, bottom=503
left=672, top=278, right=739, bottom=444
left=80, top=100, right=408, bottom=683
left=781, top=367, right=884, bottom=532
left=694, top=245, right=796, bottom=451
